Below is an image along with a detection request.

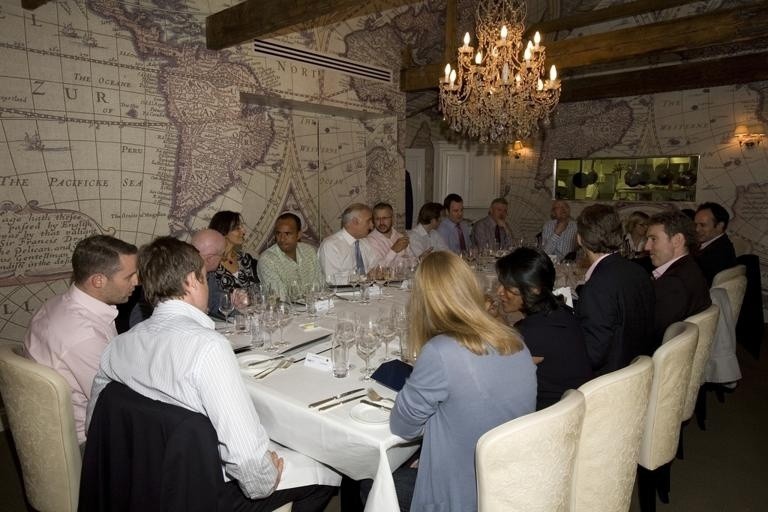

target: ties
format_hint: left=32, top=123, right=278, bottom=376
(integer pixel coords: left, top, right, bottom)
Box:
left=355, top=240, right=365, bottom=275
left=495, top=224, right=500, bottom=242
left=456, top=224, right=466, bottom=250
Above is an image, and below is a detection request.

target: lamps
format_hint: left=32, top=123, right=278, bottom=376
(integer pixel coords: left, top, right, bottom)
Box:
left=729, top=122, right=768, bottom=154
left=503, top=139, right=536, bottom=162
left=435, top=1, right=565, bottom=152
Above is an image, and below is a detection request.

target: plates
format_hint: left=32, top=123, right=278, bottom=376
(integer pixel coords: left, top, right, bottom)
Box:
left=350, top=402, right=394, bottom=425
left=238, top=355, right=273, bottom=375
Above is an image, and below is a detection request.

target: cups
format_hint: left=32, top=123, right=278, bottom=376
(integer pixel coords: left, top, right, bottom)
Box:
left=619, top=243, right=641, bottom=260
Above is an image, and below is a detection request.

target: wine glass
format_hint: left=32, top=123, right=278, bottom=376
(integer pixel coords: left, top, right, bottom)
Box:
left=455, top=236, right=575, bottom=298
left=218, top=272, right=337, bottom=354
left=347, top=257, right=419, bottom=306
left=331, top=302, right=417, bottom=383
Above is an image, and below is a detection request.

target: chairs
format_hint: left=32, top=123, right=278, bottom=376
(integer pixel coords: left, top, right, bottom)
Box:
left=703, top=266, right=747, bottom=385
left=0, top=338, right=84, bottom=512
left=675, top=304, right=727, bottom=461
left=636, top=320, right=700, bottom=510
left=472, top=388, right=588, bottom=512
left=572, top=353, right=655, bottom=512
left=76, top=381, right=230, bottom=511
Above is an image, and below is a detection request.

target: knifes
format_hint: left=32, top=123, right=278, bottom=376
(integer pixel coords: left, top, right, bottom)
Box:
left=248, top=355, right=284, bottom=366
left=359, top=398, right=393, bottom=411
left=309, top=388, right=364, bottom=408
left=318, top=393, right=366, bottom=412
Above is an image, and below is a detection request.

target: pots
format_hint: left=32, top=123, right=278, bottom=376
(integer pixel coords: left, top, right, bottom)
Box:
left=573, top=159, right=587, bottom=188
left=587, top=159, right=597, bottom=185
left=623, top=158, right=696, bottom=187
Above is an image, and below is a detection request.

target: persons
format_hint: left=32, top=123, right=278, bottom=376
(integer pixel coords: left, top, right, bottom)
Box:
left=26, top=235, right=138, bottom=455
left=130, top=210, right=259, bottom=330
left=484, top=244, right=596, bottom=413
left=361, top=250, right=538, bottom=509
left=473, top=198, right=512, bottom=250
left=84, top=236, right=340, bottom=511
left=257, top=213, right=323, bottom=301
left=318, top=202, right=434, bottom=288
left=624, top=201, right=734, bottom=345
left=403, top=194, right=471, bottom=261
left=570, top=203, right=655, bottom=379
left=540, top=201, right=578, bottom=262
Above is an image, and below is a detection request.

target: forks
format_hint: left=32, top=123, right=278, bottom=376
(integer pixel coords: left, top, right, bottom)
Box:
left=253, top=356, right=295, bottom=379
left=366, top=386, right=395, bottom=403
